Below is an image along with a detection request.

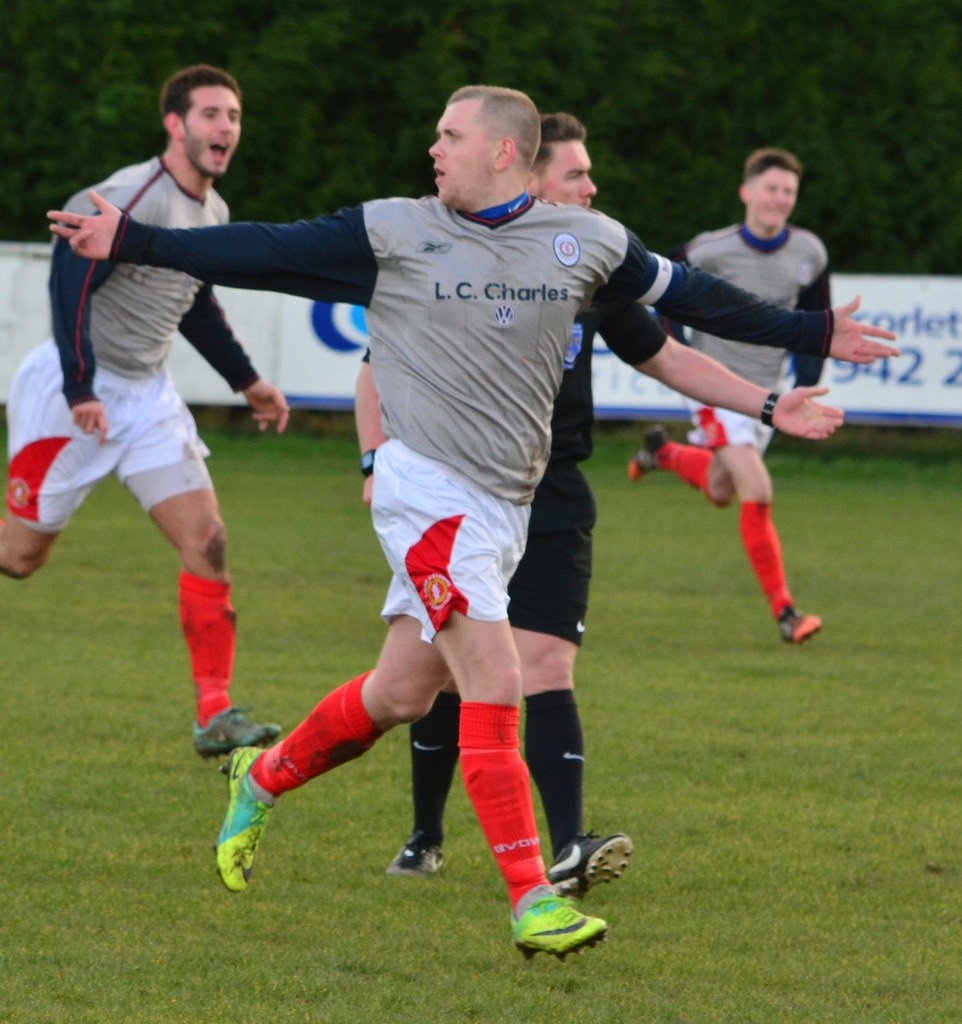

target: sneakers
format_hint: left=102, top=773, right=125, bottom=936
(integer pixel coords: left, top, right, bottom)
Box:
left=626, top=425, right=669, bottom=482
left=547, top=829, right=634, bottom=905
left=212, top=746, right=275, bottom=894
left=778, top=604, right=822, bottom=644
left=386, top=836, right=442, bottom=877
left=511, top=897, right=609, bottom=962
left=196, top=706, right=282, bottom=761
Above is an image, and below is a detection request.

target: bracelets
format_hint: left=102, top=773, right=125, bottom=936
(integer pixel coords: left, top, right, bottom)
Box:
left=762, top=393, right=779, bottom=427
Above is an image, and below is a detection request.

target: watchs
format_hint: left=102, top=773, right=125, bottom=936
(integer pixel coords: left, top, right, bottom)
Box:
left=360, top=449, right=376, bottom=476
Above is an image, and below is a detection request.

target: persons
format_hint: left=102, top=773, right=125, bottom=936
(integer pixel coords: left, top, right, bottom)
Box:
left=46, top=85, right=899, bottom=961
left=628, top=149, right=833, bottom=642
left=0, top=66, right=292, bottom=757
left=355, top=118, right=844, bottom=897
